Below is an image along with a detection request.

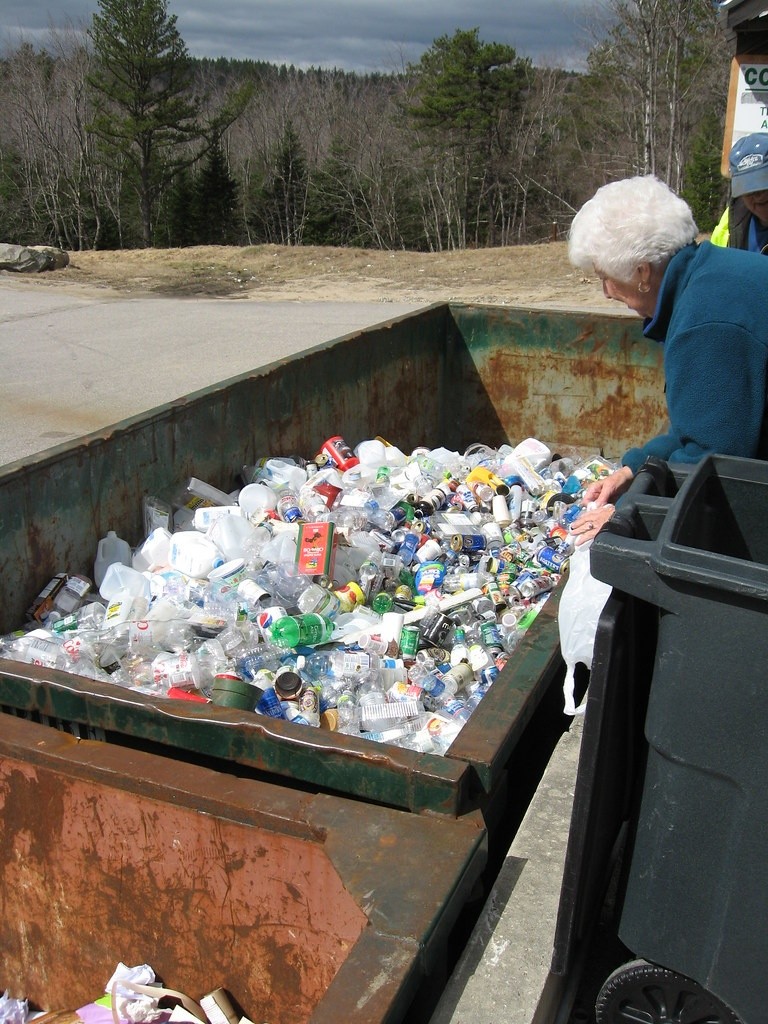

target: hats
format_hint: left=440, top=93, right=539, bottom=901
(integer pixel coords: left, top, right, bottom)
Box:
left=729, top=132, right=768, bottom=198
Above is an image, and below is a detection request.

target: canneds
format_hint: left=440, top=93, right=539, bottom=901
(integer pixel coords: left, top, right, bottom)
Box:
left=166, top=453, right=580, bottom=717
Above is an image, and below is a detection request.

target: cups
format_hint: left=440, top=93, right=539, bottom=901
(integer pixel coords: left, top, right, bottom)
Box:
left=211, top=676, right=264, bottom=713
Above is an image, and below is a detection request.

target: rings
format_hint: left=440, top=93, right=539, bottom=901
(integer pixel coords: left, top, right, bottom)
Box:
left=589, top=522, right=593, bottom=529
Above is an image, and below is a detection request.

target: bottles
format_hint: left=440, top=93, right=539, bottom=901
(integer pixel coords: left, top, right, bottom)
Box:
left=1, top=432, right=620, bottom=754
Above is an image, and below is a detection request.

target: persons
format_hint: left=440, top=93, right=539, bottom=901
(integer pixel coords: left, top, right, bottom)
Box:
left=710, top=132, right=768, bottom=258
left=568, top=175, right=768, bottom=548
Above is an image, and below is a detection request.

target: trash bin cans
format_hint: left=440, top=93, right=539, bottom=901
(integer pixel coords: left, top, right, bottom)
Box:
left=551, top=449, right=768, bottom=1023
